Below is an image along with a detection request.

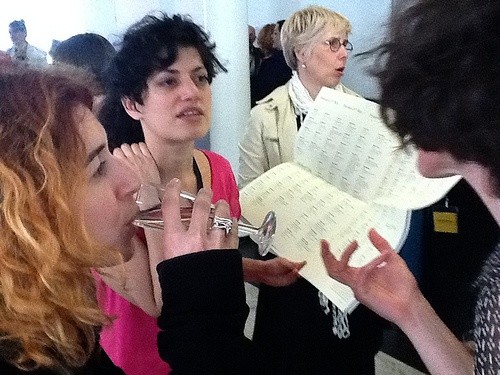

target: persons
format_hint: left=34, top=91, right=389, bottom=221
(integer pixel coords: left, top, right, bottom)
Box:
left=237, top=6, right=500, bottom=375
left=320, top=0, right=500, bottom=375
left=0, top=16, right=144, bottom=156
left=91, top=9, right=241, bottom=375
left=0, top=62, right=251, bottom=375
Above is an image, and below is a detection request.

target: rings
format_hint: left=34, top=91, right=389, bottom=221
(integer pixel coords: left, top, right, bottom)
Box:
left=211, top=213, right=233, bottom=235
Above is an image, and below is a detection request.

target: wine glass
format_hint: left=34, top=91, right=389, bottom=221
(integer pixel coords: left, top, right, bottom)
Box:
left=131, top=180, right=277, bottom=257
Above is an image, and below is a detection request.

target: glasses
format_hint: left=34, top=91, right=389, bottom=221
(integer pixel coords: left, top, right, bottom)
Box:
left=326, top=37, right=353, bottom=52
